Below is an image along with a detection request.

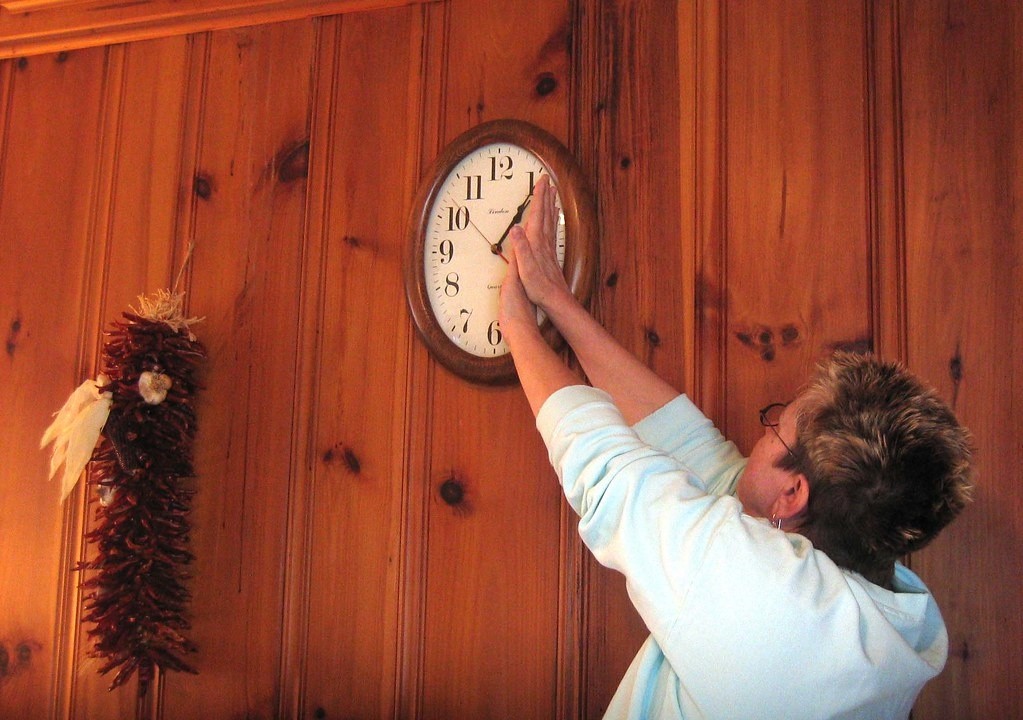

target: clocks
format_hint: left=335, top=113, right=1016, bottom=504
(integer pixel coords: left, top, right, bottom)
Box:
left=401, top=118, right=598, bottom=387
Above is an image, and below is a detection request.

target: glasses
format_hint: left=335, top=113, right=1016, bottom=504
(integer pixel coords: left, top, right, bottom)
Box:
left=759, top=401, right=807, bottom=473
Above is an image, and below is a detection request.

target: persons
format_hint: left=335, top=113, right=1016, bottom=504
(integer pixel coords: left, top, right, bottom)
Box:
left=499, top=173, right=975, bottom=720
